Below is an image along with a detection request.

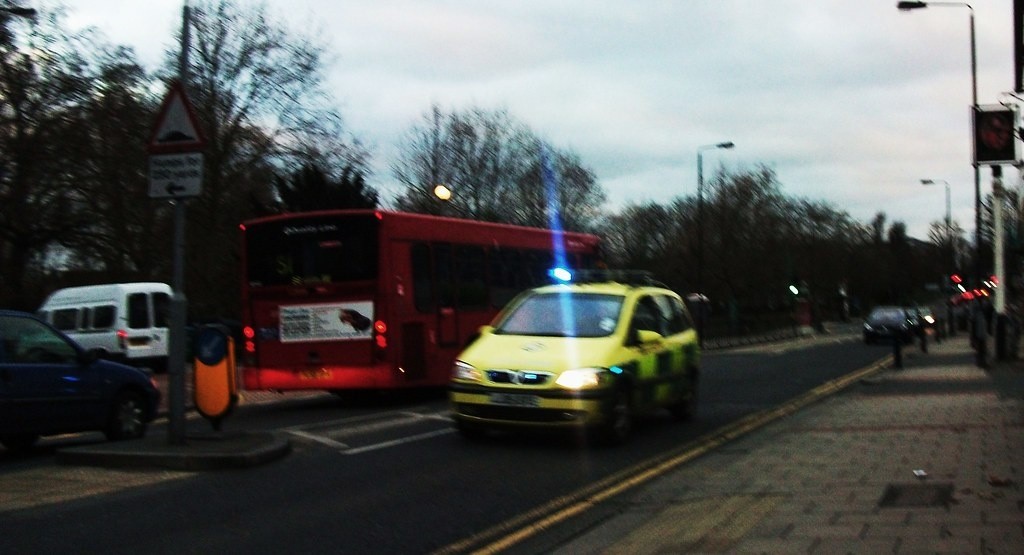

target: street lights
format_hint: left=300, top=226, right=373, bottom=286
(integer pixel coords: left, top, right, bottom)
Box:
left=896, top=0, right=984, bottom=279
left=919, top=179, right=956, bottom=338
left=693, top=140, right=736, bottom=353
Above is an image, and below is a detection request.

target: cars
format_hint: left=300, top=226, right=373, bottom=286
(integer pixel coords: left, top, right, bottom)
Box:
left=441, top=280, right=700, bottom=448
left=0, top=308, right=162, bottom=454
left=866, top=303, right=936, bottom=346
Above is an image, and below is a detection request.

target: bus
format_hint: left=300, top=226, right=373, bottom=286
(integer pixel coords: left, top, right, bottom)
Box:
left=233, top=205, right=610, bottom=407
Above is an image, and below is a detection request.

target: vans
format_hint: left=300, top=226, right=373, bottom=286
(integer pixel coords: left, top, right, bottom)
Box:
left=17, top=281, right=172, bottom=373
